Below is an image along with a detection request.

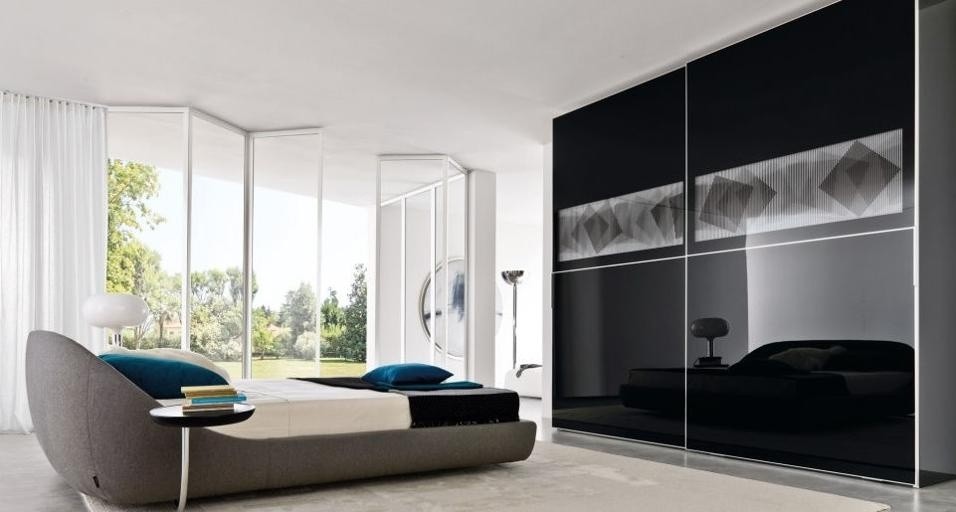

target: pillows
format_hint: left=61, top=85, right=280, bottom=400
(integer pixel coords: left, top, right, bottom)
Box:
left=97, top=347, right=230, bottom=396
left=360, top=360, right=452, bottom=388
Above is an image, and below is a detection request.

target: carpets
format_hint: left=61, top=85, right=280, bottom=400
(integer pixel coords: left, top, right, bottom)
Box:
left=553, top=405, right=915, bottom=471
left=76, top=443, right=890, bottom=511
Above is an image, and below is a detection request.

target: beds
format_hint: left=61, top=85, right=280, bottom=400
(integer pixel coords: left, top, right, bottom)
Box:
left=619, top=339, right=915, bottom=434
left=26, top=331, right=536, bottom=501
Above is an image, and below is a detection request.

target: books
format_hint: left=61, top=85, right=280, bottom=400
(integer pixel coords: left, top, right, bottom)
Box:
left=180, top=385, right=248, bottom=414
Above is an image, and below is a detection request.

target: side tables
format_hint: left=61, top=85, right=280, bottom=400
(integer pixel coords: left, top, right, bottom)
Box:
left=148, top=405, right=254, bottom=512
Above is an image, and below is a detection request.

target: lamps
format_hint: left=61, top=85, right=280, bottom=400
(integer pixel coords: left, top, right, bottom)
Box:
left=691, top=317, right=730, bottom=366
left=85, top=291, right=149, bottom=345
